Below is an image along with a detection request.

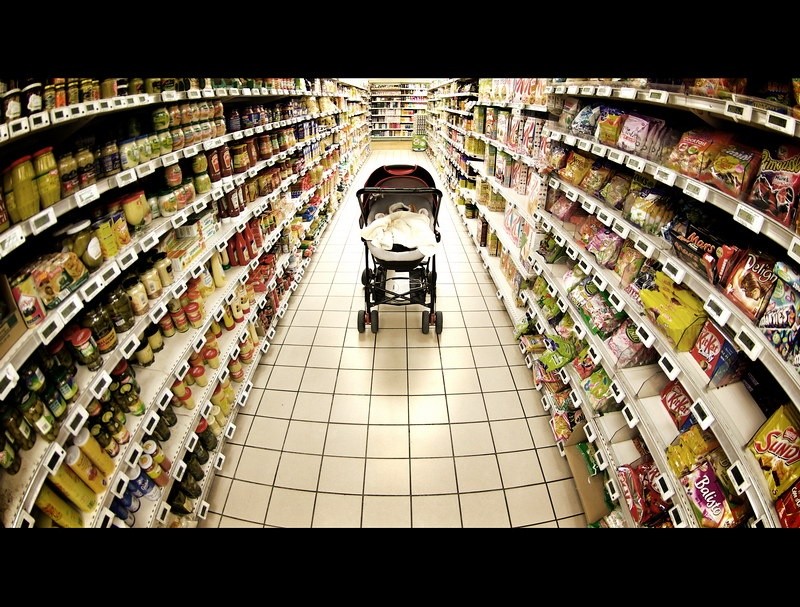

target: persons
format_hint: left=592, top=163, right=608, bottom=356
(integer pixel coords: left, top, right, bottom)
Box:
left=375, top=202, right=430, bottom=249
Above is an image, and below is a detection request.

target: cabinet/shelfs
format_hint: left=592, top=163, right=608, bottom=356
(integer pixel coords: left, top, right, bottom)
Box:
left=1, top=70, right=370, bottom=531
left=456, top=100, right=547, bottom=326
left=367, top=80, right=429, bottom=142
left=425, top=80, right=479, bottom=206
left=512, top=86, right=800, bottom=529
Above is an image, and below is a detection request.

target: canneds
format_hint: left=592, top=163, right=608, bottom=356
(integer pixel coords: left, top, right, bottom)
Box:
left=0, top=75, right=295, bottom=529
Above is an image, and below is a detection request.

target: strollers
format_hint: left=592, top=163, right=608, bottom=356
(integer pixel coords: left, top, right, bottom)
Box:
left=355, top=164, right=445, bottom=336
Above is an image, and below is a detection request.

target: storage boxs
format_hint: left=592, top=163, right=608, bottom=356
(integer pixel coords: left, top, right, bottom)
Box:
left=463, top=78, right=553, bottom=307
